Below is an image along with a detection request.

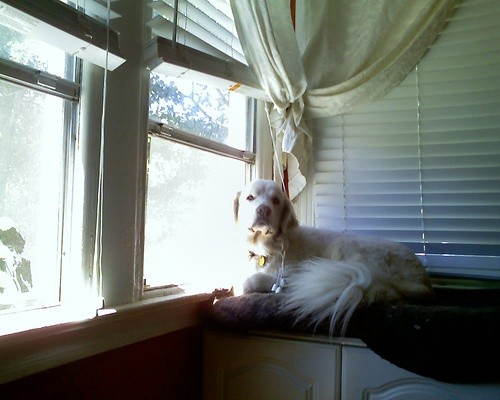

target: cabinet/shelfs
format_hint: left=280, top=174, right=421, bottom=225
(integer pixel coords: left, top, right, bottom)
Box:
left=199, top=330, right=500, bottom=400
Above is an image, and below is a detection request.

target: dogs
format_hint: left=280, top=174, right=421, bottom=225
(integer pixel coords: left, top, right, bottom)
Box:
left=234, top=179, right=430, bottom=342
